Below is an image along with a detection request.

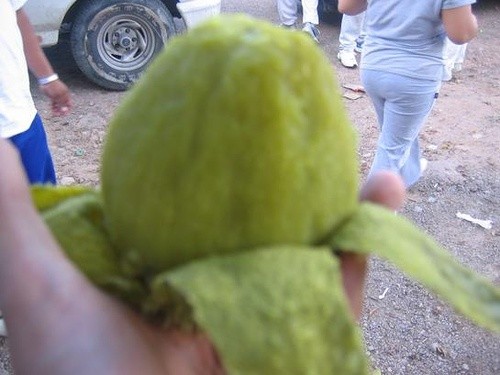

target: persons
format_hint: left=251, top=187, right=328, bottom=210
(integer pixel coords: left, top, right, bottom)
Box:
left=338, top=0, right=478, bottom=189
left=338, top=10, right=366, bottom=68
left=278, top=0, right=321, bottom=44
left=442, top=35, right=467, bottom=81
left=0, top=137, right=407, bottom=375
left=0, top=0, right=72, bottom=186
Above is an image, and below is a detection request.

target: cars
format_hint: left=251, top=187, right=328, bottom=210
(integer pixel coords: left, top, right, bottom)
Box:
left=22, top=0, right=223, bottom=92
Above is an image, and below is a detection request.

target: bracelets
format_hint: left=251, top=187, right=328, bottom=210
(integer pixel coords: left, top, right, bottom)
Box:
left=39, top=74, right=58, bottom=85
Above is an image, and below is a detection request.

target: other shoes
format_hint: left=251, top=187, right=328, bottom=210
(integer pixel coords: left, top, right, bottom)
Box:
left=420, top=159, right=427, bottom=174
left=454, top=63, right=461, bottom=73
left=441, top=72, right=452, bottom=82
left=302, top=23, right=319, bottom=44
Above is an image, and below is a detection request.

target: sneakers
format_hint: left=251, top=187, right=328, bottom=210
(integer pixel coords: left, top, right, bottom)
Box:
left=337, top=49, right=358, bottom=68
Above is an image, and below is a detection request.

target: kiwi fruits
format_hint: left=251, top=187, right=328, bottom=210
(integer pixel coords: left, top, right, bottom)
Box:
left=26, top=12, right=500, bottom=375
